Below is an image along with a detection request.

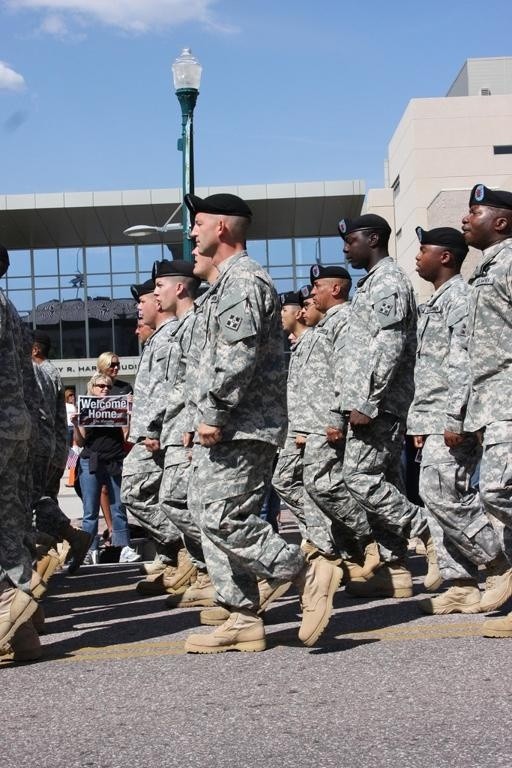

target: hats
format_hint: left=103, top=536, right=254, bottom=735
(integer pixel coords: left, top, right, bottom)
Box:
left=184, top=193, right=252, bottom=216
left=416, top=226, right=469, bottom=251
left=131, top=278, right=155, bottom=296
left=32, top=329, right=51, bottom=344
left=469, top=183, right=512, bottom=209
left=152, top=258, right=200, bottom=283
left=277, top=264, right=352, bottom=310
left=338, top=213, right=391, bottom=237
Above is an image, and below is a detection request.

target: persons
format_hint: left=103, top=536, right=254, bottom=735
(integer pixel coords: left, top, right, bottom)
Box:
left=119, top=194, right=344, bottom=653
left=0, top=184, right=90, bottom=662
left=280, top=183, right=512, bottom=639
left=70, top=352, right=142, bottom=564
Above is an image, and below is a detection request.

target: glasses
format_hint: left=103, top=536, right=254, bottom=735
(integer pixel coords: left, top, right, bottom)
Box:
left=110, top=361, right=119, bottom=367
left=94, top=383, right=112, bottom=390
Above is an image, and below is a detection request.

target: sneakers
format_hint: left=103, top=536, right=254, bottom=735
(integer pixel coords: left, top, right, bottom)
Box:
left=83, top=549, right=98, bottom=564
left=118, top=546, right=141, bottom=562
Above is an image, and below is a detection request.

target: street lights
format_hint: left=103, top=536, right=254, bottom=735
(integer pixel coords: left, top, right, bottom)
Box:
left=123, top=49, right=202, bottom=281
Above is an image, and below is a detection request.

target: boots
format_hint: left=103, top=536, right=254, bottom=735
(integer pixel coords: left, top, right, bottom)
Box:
left=0, top=575, right=39, bottom=651
left=482, top=610, right=512, bottom=637
left=343, top=530, right=444, bottom=597
left=0, top=618, right=43, bottom=666
left=479, top=564, right=512, bottom=611
left=136, top=547, right=292, bottom=626
left=69, top=528, right=94, bottom=572
left=419, top=585, right=483, bottom=614
left=184, top=610, right=267, bottom=655
left=30, top=537, right=72, bottom=622
left=292, top=558, right=345, bottom=646
